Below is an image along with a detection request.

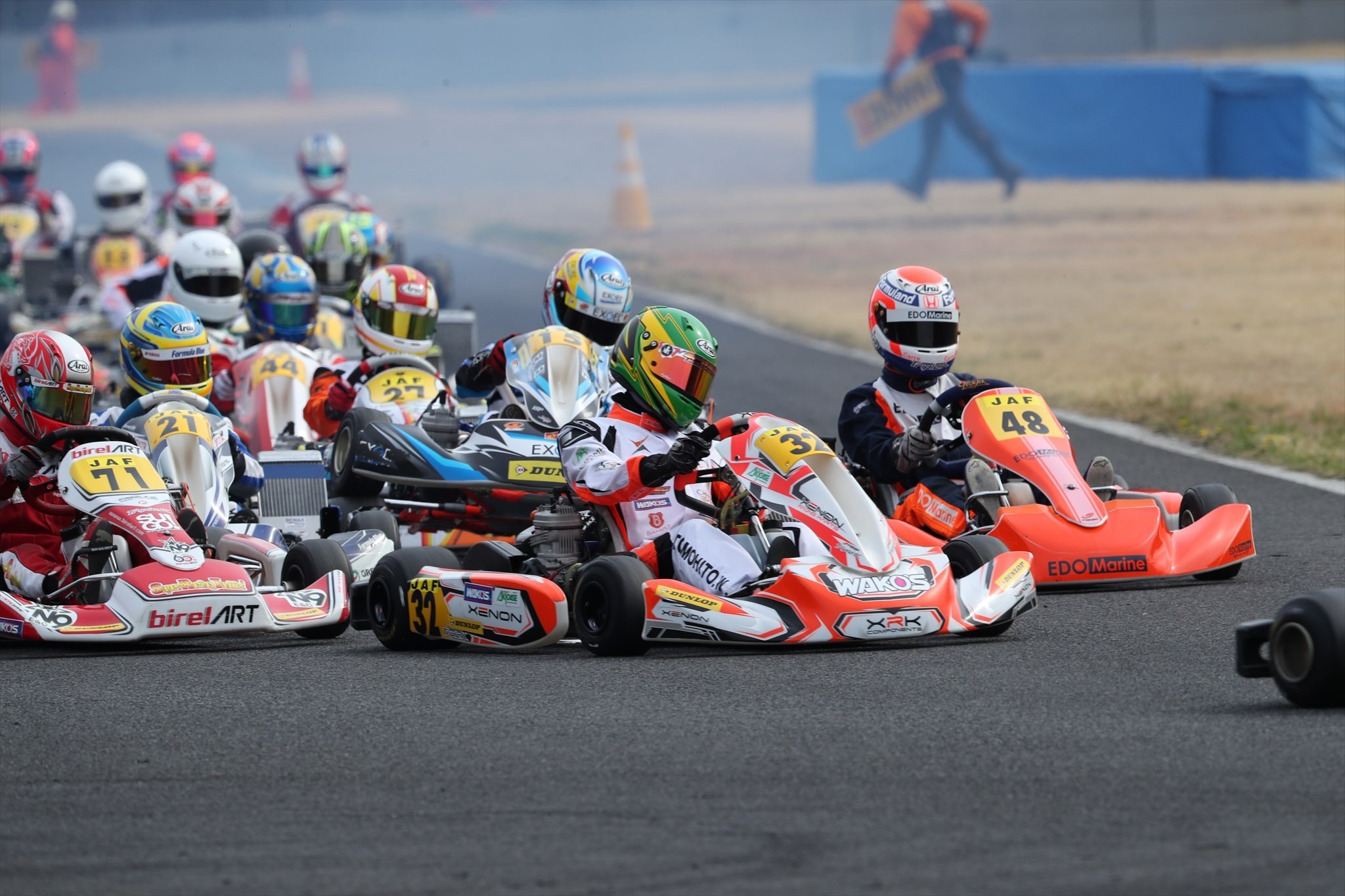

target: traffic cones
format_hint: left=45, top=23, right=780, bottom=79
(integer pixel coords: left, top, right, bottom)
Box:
left=612, top=117, right=656, bottom=236
left=283, top=42, right=314, bottom=103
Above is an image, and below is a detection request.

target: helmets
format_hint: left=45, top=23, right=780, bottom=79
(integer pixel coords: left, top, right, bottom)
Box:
left=119, top=300, right=214, bottom=401
left=166, top=229, right=246, bottom=328
left=609, top=305, right=719, bottom=431
left=0, top=126, right=41, bottom=174
left=542, top=248, right=633, bottom=351
left=296, top=132, right=349, bottom=195
left=78, top=161, right=166, bottom=297
left=227, top=228, right=320, bottom=346
left=164, top=132, right=234, bottom=230
left=353, top=264, right=439, bottom=359
left=868, top=265, right=958, bottom=378
left=0, top=330, right=96, bottom=452
left=293, top=201, right=397, bottom=299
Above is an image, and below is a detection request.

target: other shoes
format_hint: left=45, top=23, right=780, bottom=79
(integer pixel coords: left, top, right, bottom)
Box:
left=228, top=509, right=260, bottom=524
left=1006, top=163, right=1022, bottom=201
left=897, top=173, right=927, bottom=202
left=498, top=404, right=529, bottom=421
left=177, top=508, right=209, bottom=556
left=963, top=459, right=1003, bottom=524
left=48, top=517, right=114, bottom=605
left=763, top=535, right=799, bottom=587
left=1084, top=456, right=1114, bottom=502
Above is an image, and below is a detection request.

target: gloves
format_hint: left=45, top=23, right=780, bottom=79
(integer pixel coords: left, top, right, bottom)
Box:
left=43, top=190, right=76, bottom=251
left=326, top=379, right=358, bottom=413
left=900, top=427, right=938, bottom=460
left=228, top=433, right=246, bottom=486
left=214, top=369, right=237, bottom=402
left=641, top=436, right=710, bottom=489
left=479, top=332, right=522, bottom=386
left=4, top=445, right=46, bottom=484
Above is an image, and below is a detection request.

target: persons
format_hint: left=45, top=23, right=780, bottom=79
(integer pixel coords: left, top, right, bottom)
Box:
left=211, top=252, right=347, bottom=451
left=0, top=330, right=208, bottom=605
left=837, top=264, right=1114, bottom=542
left=0, top=129, right=395, bottom=331
left=302, top=264, right=524, bottom=470
left=29, top=0, right=78, bottom=116
left=89, top=301, right=265, bottom=523
left=882, top=0, right=1018, bottom=199
left=455, top=249, right=633, bottom=421
left=556, top=305, right=800, bottom=597
